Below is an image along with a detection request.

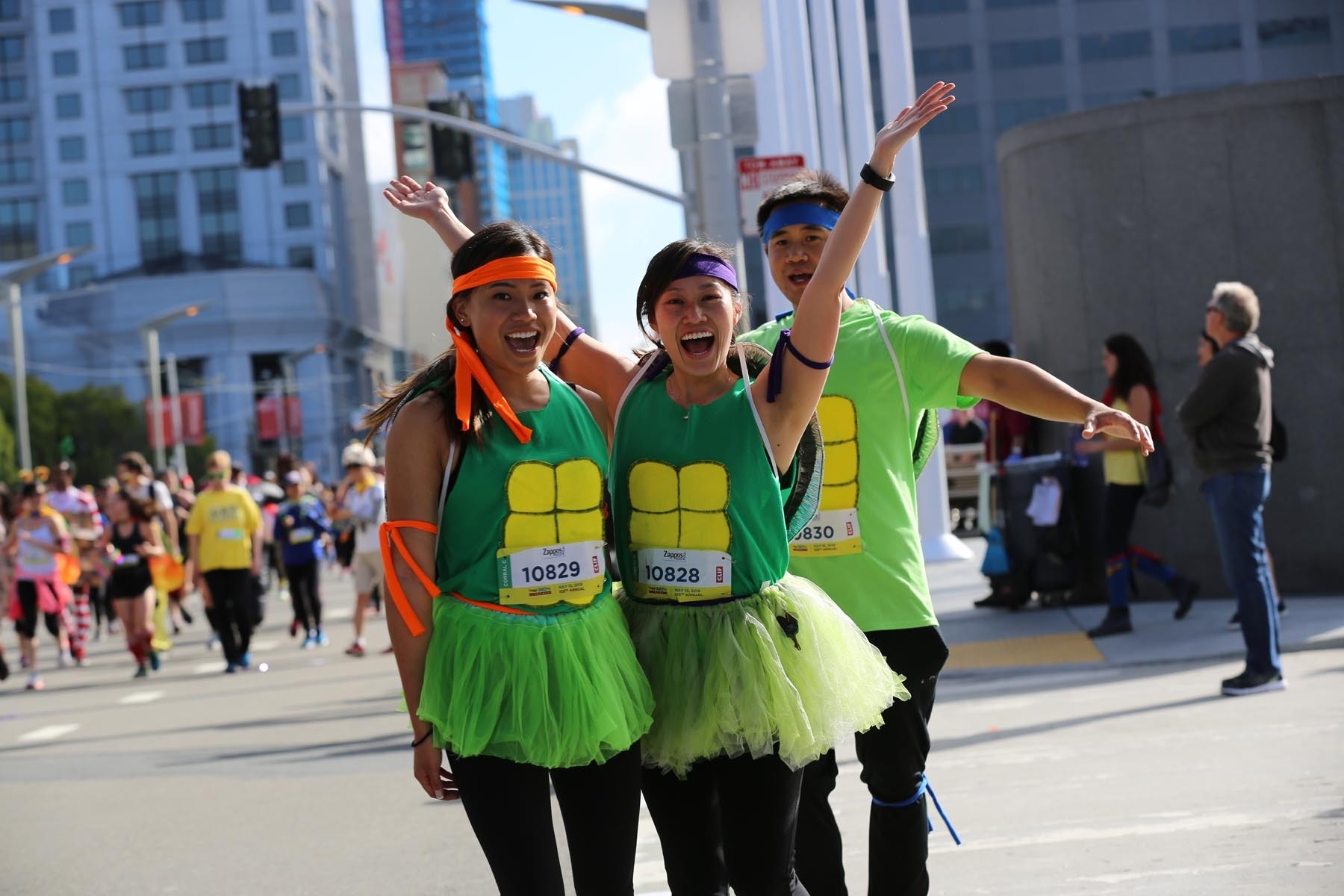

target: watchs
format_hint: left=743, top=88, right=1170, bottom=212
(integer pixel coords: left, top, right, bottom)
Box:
left=860, top=161, right=895, bottom=191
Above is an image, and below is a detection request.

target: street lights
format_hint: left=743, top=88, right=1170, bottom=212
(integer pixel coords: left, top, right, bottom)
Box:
left=0, top=245, right=84, bottom=470
left=280, top=345, right=326, bottom=454
left=140, top=301, right=214, bottom=477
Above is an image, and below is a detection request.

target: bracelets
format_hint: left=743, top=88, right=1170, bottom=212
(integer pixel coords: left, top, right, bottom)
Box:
left=1010, top=446, right=1023, bottom=454
left=411, top=728, right=433, bottom=747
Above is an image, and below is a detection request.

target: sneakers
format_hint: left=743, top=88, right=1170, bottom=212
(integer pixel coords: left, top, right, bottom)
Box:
left=1221, top=671, right=1286, bottom=695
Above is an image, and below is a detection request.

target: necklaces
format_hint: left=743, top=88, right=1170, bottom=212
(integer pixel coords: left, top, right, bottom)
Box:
left=667, top=375, right=732, bottom=420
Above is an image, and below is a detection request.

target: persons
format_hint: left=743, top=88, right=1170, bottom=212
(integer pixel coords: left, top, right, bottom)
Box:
left=386, top=220, right=654, bottom=896
left=382, top=79, right=955, bottom=896
left=0, top=440, right=394, bottom=690
left=729, top=174, right=1157, bottom=896
left=943, top=337, right=1039, bottom=607
left=1175, top=283, right=1289, bottom=696
left=1074, top=333, right=1201, bottom=637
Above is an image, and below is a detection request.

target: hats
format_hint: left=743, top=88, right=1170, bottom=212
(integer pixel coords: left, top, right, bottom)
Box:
left=283, top=471, right=303, bottom=485
left=342, top=443, right=377, bottom=468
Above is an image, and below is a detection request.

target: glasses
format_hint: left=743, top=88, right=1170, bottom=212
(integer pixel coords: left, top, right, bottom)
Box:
left=204, top=471, right=231, bottom=479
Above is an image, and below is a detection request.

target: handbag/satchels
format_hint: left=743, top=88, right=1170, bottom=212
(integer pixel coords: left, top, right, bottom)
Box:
left=980, top=528, right=1010, bottom=574
left=1144, top=438, right=1173, bottom=509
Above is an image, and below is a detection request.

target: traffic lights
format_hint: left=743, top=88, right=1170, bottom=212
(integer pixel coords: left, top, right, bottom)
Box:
left=428, top=97, right=469, bottom=182
left=236, top=77, right=283, bottom=173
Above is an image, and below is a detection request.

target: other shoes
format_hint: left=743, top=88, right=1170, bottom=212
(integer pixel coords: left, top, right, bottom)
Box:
left=226, top=662, right=235, bottom=672
left=302, top=637, right=314, bottom=649
left=346, top=645, right=363, bottom=654
left=1226, top=615, right=1241, bottom=629
left=1277, top=600, right=1289, bottom=617
left=1087, top=622, right=1132, bottom=637
left=21, top=656, right=30, bottom=667
left=150, top=652, right=159, bottom=670
left=383, top=647, right=393, bottom=654
left=78, top=659, right=97, bottom=666
left=1175, top=583, right=1200, bottom=619
left=135, top=668, right=146, bottom=676
left=291, top=618, right=298, bottom=636
left=316, top=634, right=325, bottom=644
left=239, top=653, right=248, bottom=666
left=26, top=676, right=43, bottom=690
left=974, top=594, right=1028, bottom=605
left=59, top=654, right=72, bottom=667
